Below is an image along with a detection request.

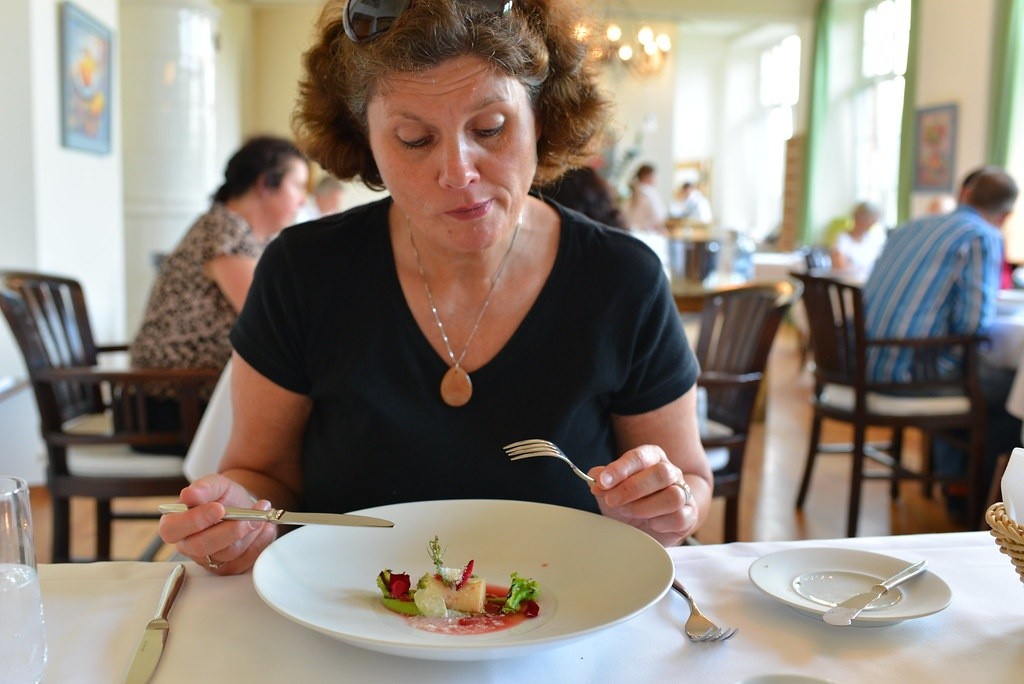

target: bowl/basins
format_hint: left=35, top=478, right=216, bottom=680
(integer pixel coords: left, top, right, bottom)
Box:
left=997, top=289, right=1024, bottom=313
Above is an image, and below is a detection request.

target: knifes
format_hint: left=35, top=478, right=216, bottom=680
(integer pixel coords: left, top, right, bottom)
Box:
left=125, top=564, right=186, bottom=684
left=823, top=558, right=931, bottom=625
left=158, top=504, right=395, bottom=527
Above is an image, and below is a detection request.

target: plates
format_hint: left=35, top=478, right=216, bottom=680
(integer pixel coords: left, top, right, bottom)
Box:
left=749, top=547, right=952, bottom=626
left=253, top=500, right=675, bottom=660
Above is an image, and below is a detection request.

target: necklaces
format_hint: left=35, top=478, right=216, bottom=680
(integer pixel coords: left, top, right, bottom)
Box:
left=406, top=214, right=522, bottom=406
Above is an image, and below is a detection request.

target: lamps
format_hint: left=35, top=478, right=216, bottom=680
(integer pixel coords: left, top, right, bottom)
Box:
left=568, top=0, right=673, bottom=80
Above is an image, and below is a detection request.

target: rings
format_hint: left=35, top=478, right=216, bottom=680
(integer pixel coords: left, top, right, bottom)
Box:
left=207, top=555, right=227, bottom=569
left=675, top=480, right=692, bottom=503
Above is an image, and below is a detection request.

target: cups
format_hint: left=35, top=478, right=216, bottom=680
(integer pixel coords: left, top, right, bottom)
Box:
left=0, top=476, right=48, bottom=684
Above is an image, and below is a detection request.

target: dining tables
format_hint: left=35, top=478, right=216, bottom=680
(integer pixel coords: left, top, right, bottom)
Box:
left=0, top=531, right=1024, bottom=684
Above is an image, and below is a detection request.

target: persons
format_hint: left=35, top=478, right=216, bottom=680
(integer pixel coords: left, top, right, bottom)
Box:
left=834, top=200, right=886, bottom=282
left=129, top=137, right=311, bottom=461
left=631, top=166, right=665, bottom=231
left=541, top=165, right=633, bottom=231
left=158, top=0, right=715, bottom=576
left=678, top=182, right=711, bottom=223
left=298, top=174, right=341, bottom=222
left=838, top=167, right=1024, bottom=510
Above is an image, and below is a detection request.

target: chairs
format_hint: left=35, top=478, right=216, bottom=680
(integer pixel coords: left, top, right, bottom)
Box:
left=0, top=269, right=223, bottom=565
left=674, top=271, right=993, bottom=547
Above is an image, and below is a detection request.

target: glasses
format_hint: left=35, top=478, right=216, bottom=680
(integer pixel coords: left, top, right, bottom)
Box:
left=331, top=0, right=523, bottom=46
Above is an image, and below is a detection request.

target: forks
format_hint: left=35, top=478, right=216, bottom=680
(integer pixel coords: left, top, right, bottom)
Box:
left=671, top=577, right=740, bottom=642
left=504, top=439, right=595, bottom=485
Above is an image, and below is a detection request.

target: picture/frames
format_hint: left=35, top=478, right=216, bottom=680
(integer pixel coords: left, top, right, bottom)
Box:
left=905, top=104, right=959, bottom=199
left=54, top=0, right=114, bottom=155
